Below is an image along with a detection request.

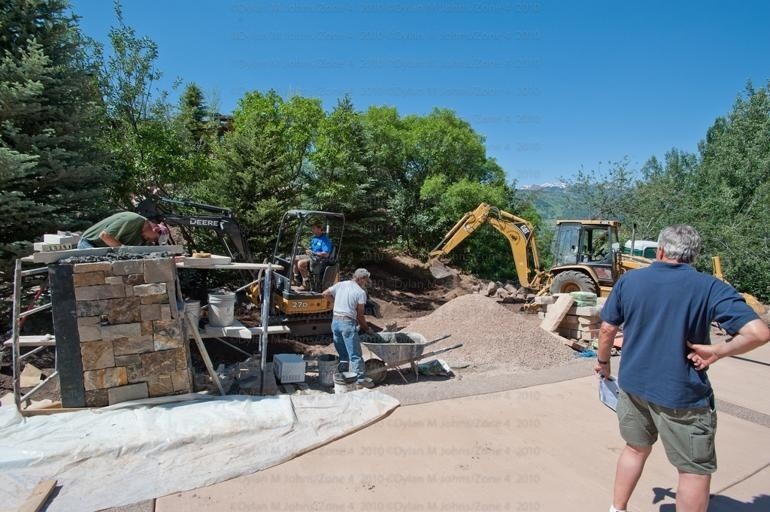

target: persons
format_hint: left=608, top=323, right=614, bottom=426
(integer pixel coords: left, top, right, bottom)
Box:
left=292, top=220, right=332, bottom=292
left=594, top=223, right=770, bottom=512
left=321, top=267, right=375, bottom=389
left=77, top=211, right=170, bottom=250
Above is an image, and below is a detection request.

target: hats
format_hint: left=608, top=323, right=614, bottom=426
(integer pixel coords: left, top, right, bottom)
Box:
left=157, top=224, right=169, bottom=245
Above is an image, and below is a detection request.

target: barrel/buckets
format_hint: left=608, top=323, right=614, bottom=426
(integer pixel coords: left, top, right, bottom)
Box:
left=207, top=290, right=236, bottom=327
left=318, top=354, right=340, bottom=387
left=184, top=298, right=200, bottom=338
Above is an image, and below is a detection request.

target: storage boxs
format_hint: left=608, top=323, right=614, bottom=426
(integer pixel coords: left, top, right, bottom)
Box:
left=273, top=353, right=307, bottom=384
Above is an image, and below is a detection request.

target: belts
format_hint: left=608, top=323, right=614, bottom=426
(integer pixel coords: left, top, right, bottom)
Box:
left=334, top=316, right=351, bottom=320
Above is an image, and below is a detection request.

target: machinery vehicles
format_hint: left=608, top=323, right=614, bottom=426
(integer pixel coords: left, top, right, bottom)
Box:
left=135, top=188, right=378, bottom=346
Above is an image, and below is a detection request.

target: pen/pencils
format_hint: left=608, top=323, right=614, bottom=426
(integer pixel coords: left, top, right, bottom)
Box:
left=600, top=373, right=614, bottom=381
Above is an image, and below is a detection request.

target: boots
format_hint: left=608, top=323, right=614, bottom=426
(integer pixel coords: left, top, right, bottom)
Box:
left=292, top=276, right=310, bottom=291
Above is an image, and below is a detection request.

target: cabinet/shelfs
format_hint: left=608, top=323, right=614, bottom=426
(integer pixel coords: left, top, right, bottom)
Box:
left=6, top=255, right=291, bottom=415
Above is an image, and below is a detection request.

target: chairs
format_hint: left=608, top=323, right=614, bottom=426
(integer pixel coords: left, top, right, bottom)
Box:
left=311, top=245, right=337, bottom=290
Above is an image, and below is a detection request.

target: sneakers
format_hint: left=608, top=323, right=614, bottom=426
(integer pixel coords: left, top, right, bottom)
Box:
left=357, top=381, right=375, bottom=389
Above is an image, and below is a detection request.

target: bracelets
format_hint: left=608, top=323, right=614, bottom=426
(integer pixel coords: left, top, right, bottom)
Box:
left=597, top=358, right=610, bottom=365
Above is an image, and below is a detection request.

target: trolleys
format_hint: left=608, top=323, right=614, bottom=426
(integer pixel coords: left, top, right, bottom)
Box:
left=358, top=331, right=463, bottom=386
